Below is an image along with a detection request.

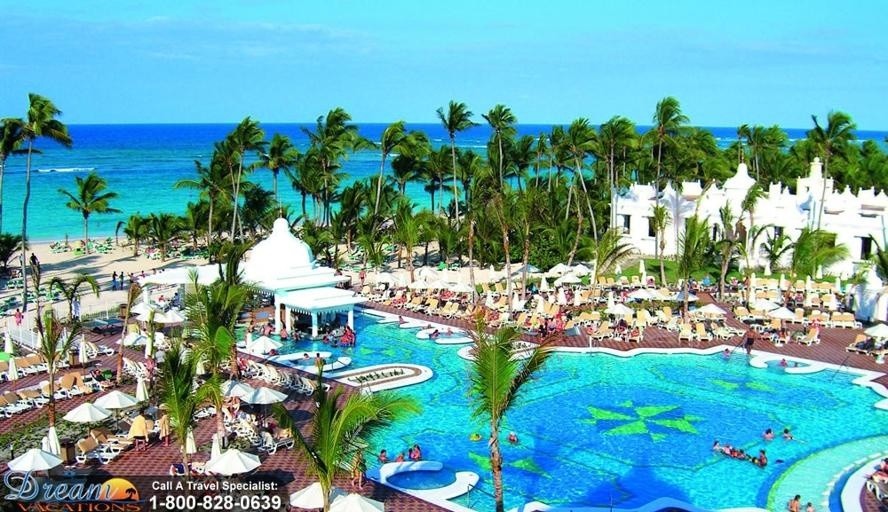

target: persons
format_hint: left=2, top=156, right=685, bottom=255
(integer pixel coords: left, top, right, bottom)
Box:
left=144, top=413, right=154, bottom=430
left=79, top=239, right=85, bottom=247
left=363, top=259, right=888, bottom=354
left=157, top=411, right=172, bottom=447
left=315, top=352, right=321, bottom=359
left=14, top=307, right=21, bottom=326
left=508, top=432, right=518, bottom=442
left=72, top=297, right=80, bottom=316
left=159, top=294, right=165, bottom=302
left=270, top=349, right=275, bottom=356
left=239, top=357, right=250, bottom=372
left=125, top=409, right=151, bottom=452
left=280, top=326, right=287, bottom=341
left=127, top=273, right=137, bottom=284
left=226, top=358, right=238, bottom=380
left=171, top=232, right=239, bottom=253
left=408, top=448, right=414, bottom=459
left=247, top=319, right=254, bottom=332
left=119, top=271, right=125, bottom=291
left=111, top=270, right=119, bottom=291
left=778, top=358, right=786, bottom=366
left=761, top=428, right=773, bottom=441
left=145, top=247, right=150, bottom=259
left=322, top=325, right=355, bottom=347
left=18, top=253, right=22, bottom=265
left=303, top=353, right=310, bottom=360
left=469, top=433, right=481, bottom=441
left=152, top=268, right=156, bottom=275
left=65, top=242, right=72, bottom=251
left=394, top=452, right=405, bottom=463
left=146, top=354, right=154, bottom=375
left=172, top=292, right=179, bottom=306
left=378, top=449, right=389, bottom=461
left=782, top=429, right=793, bottom=443
left=9, top=442, right=14, bottom=457
left=805, top=502, right=815, bottom=512
left=349, top=450, right=360, bottom=488
left=155, top=346, right=164, bottom=369
left=139, top=270, right=146, bottom=279
left=89, top=370, right=110, bottom=387
left=221, top=396, right=294, bottom=448
left=410, top=444, right=422, bottom=461
left=711, top=440, right=768, bottom=470
left=784, top=495, right=801, bottom=512
left=863, top=469, right=888, bottom=479
left=294, top=330, right=298, bottom=341
left=30, top=252, right=38, bottom=266
left=356, top=453, right=368, bottom=490
left=264, top=323, right=275, bottom=337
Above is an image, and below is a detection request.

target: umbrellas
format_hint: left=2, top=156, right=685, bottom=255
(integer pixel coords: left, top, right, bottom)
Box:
left=61, top=403, right=113, bottom=439
left=4, top=332, right=13, bottom=356
left=204, top=448, right=262, bottom=476
left=245, top=335, right=284, bottom=358
left=35, top=329, right=43, bottom=351
left=238, top=386, right=289, bottom=417
left=287, top=481, right=348, bottom=511
left=92, top=390, right=137, bottom=430
left=41, top=436, right=51, bottom=454
left=135, top=377, right=149, bottom=402
left=78, top=333, right=88, bottom=378
left=180, top=426, right=197, bottom=463
left=325, top=493, right=385, bottom=512
left=48, top=426, right=61, bottom=456
left=195, top=358, right=205, bottom=376
left=130, top=303, right=185, bottom=331
left=114, top=333, right=147, bottom=347
left=217, top=380, right=255, bottom=398
left=6, top=448, right=64, bottom=478
left=7, top=358, right=18, bottom=392
